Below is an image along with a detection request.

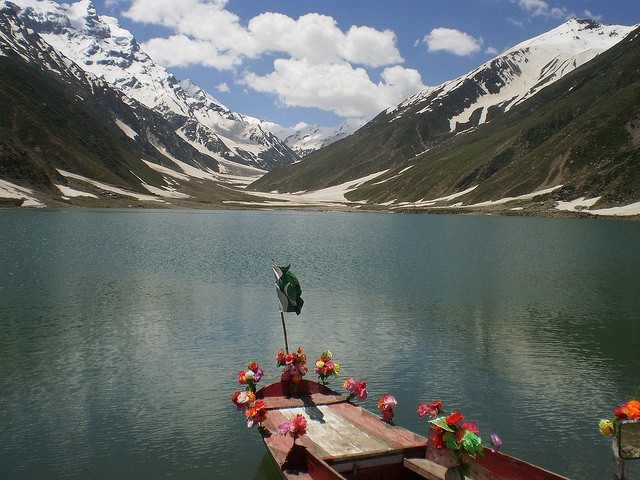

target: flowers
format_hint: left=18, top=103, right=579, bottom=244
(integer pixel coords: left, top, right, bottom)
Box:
left=245, top=401, right=267, bottom=430
left=599, top=399, right=640, bottom=439
left=276, top=347, right=308, bottom=386
left=418, top=401, right=444, bottom=422
left=377, top=395, right=398, bottom=424
left=238, top=361, right=264, bottom=389
left=431, top=412, right=502, bottom=480
left=314, top=351, right=340, bottom=386
left=343, top=378, right=368, bottom=402
left=278, top=414, right=307, bottom=436
left=232, top=392, right=257, bottom=412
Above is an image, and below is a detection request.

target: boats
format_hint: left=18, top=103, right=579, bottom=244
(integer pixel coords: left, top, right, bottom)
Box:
left=232, top=262, right=640, bottom=479
